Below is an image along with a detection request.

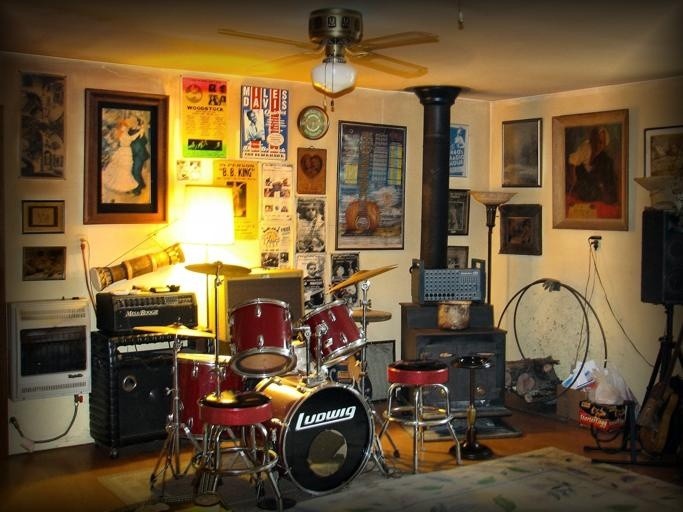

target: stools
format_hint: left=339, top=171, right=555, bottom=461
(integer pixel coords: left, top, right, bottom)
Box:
left=351, top=308, right=392, bottom=403
left=376, top=359, right=463, bottom=472
left=451, top=351, right=492, bottom=461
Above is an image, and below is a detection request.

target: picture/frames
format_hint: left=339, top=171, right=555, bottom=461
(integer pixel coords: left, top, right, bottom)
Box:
left=447, top=188, right=470, bottom=236
left=549, top=108, right=629, bottom=232
left=496, top=205, right=542, bottom=256
left=22, top=200, right=65, bottom=235
left=447, top=245, right=469, bottom=270
left=83, top=87, right=170, bottom=225
left=644, top=125, right=683, bottom=179
left=499, top=118, right=542, bottom=188
left=335, top=120, right=407, bottom=251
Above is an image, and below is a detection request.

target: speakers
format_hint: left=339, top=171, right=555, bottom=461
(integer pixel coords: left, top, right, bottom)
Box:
left=642, top=209, right=683, bottom=305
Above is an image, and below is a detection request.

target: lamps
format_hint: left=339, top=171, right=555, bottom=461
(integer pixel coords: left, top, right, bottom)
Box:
left=311, top=41, right=356, bottom=112
left=472, top=191, right=518, bottom=303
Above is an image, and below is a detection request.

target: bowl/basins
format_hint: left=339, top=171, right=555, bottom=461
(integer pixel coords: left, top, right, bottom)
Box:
left=436, top=299, right=473, bottom=332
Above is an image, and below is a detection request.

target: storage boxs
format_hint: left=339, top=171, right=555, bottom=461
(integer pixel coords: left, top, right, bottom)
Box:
left=556, top=383, right=588, bottom=422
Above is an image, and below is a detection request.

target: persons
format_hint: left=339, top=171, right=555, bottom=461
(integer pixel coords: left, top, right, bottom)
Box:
left=128, top=115, right=150, bottom=193
left=245, top=110, right=263, bottom=141
left=302, top=262, right=324, bottom=289
left=101, top=122, right=144, bottom=195
left=450, top=204, right=456, bottom=230
left=299, top=203, right=323, bottom=251
left=455, top=128, right=464, bottom=151
left=577, top=127, right=617, bottom=201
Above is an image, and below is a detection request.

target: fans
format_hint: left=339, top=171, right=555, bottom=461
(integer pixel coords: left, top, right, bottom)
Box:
left=217, top=8, right=441, bottom=79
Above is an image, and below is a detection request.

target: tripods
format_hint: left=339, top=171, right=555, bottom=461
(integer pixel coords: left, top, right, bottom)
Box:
left=634, top=306, right=682, bottom=419
left=359, top=284, right=401, bottom=459
left=149, top=337, right=222, bottom=502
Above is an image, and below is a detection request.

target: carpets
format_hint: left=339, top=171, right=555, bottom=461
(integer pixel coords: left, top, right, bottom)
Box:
left=287, top=444, right=682, bottom=512
left=98, top=454, right=396, bottom=511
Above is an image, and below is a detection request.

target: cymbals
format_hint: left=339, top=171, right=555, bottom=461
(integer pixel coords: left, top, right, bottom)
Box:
left=186, top=261, right=252, bottom=279
left=132, top=322, right=217, bottom=339
left=348, top=307, right=392, bottom=323
left=330, top=265, right=398, bottom=294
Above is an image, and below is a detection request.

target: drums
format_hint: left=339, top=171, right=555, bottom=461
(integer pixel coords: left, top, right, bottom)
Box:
left=229, top=297, right=297, bottom=378
left=324, top=364, right=372, bottom=403
left=297, top=299, right=365, bottom=365
left=89, top=243, right=186, bottom=292
left=176, top=353, right=237, bottom=442
left=241, top=374, right=376, bottom=495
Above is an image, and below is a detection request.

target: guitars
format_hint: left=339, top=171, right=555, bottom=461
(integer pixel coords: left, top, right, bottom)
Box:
left=637, top=323, right=683, bottom=456
